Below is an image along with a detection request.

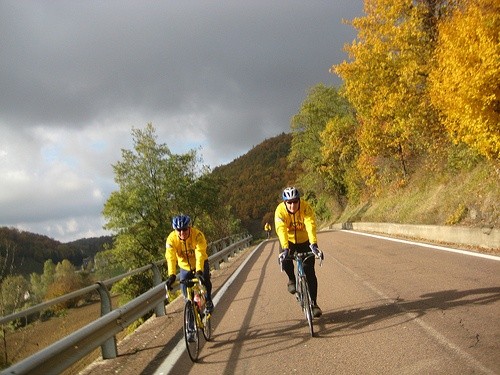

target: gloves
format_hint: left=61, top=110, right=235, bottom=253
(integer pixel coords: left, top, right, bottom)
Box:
left=195, top=271, right=206, bottom=285
left=309, top=244, right=324, bottom=260
left=165, top=274, right=177, bottom=291
left=278, top=249, right=289, bottom=264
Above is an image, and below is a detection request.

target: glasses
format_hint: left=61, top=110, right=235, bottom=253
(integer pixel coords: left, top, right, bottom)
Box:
left=176, top=227, right=189, bottom=232
left=286, top=199, right=299, bottom=205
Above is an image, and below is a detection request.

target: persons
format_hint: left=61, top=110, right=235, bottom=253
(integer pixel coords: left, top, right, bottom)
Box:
left=264, top=221, right=272, bottom=239
left=274, top=187, right=325, bottom=318
left=165, top=215, right=213, bottom=344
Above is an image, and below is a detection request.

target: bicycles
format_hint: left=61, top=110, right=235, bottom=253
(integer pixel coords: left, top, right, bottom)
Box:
left=266, top=230, right=271, bottom=240
left=280, top=251, right=322, bottom=338
left=165, top=277, right=211, bottom=363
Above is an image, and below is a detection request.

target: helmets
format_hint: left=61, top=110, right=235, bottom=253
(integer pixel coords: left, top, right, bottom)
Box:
left=282, top=187, right=300, bottom=201
left=172, top=215, right=190, bottom=229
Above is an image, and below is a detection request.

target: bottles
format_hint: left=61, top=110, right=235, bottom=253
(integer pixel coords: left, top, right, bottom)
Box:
left=194, top=294, right=200, bottom=308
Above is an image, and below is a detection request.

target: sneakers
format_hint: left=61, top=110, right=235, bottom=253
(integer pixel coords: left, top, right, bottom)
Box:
left=187, top=328, right=195, bottom=342
left=287, top=275, right=296, bottom=294
left=311, top=303, right=322, bottom=317
left=205, top=300, right=213, bottom=313
